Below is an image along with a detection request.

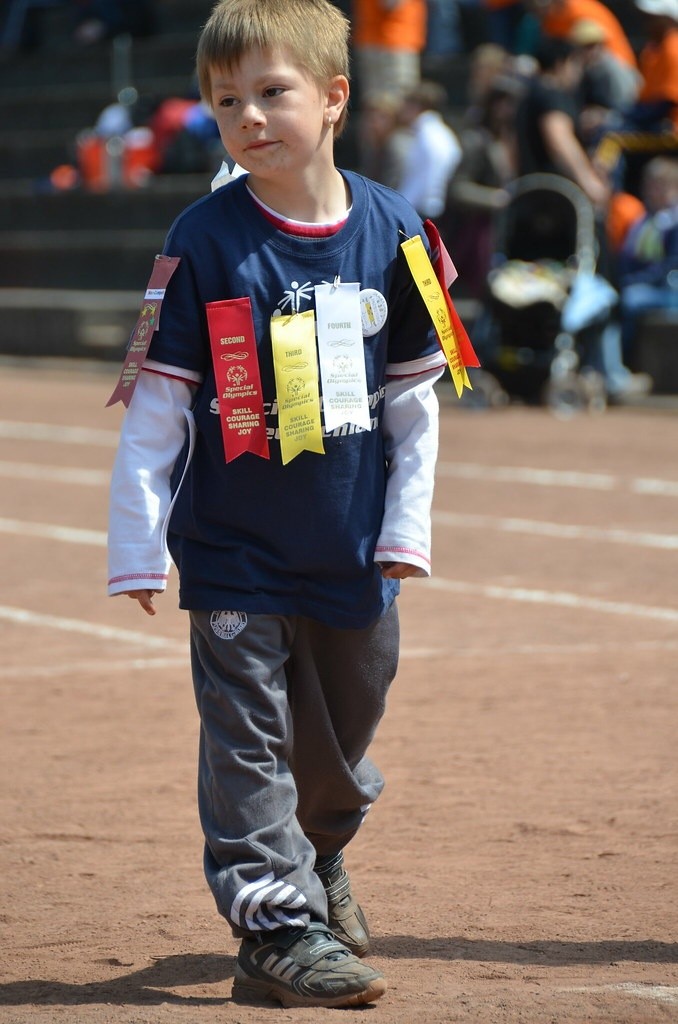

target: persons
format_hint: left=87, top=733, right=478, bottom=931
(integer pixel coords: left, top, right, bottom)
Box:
left=335, top=0, right=678, bottom=415
left=109, top=0, right=449, bottom=1010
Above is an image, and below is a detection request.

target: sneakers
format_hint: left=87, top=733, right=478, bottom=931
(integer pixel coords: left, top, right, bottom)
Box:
left=231, top=920, right=388, bottom=1007
left=311, top=849, right=372, bottom=959
